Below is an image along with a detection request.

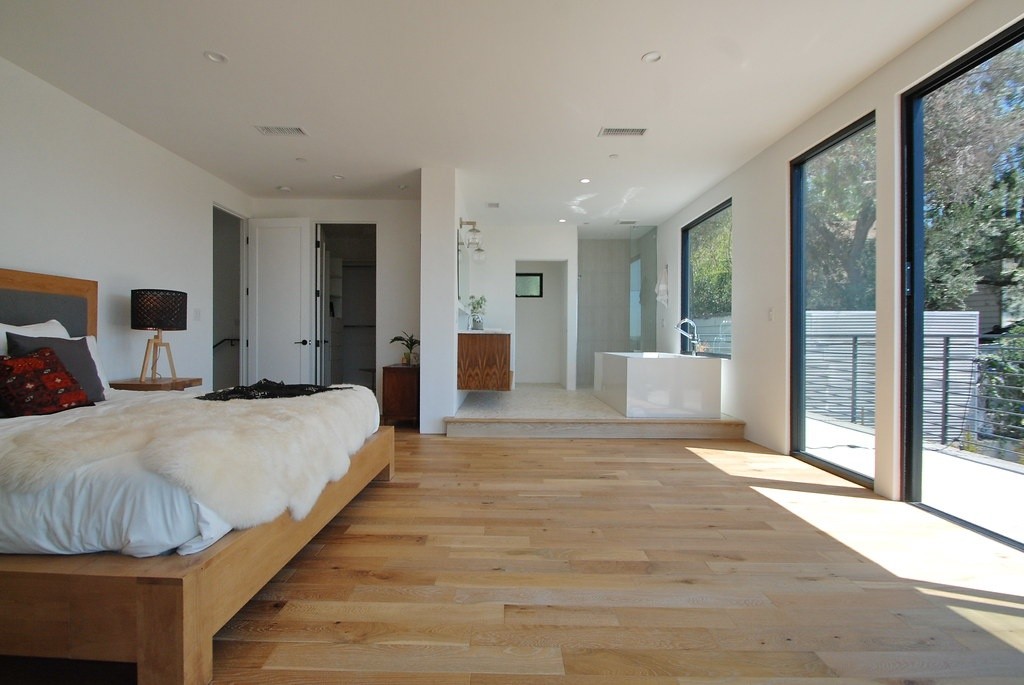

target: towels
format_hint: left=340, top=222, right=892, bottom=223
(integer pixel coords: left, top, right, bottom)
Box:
left=655, top=265, right=669, bottom=309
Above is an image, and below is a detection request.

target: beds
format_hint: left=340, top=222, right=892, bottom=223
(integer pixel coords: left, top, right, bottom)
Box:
left=0, top=267, right=396, bottom=685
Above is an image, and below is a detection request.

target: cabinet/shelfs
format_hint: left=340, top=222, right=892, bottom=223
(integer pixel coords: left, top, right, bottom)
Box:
left=382, top=364, right=420, bottom=429
left=457, top=334, right=514, bottom=392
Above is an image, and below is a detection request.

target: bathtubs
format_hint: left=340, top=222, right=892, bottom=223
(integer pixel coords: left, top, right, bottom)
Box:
left=592, top=351, right=721, bottom=419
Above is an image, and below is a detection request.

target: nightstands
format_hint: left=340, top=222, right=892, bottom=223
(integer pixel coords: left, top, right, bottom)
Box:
left=108, top=377, right=202, bottom=392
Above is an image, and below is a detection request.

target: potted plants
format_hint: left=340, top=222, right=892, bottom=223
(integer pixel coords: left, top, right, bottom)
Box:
left=467, top=294, right=487, bottom=330
left=389, top=330, right=420, bottom=366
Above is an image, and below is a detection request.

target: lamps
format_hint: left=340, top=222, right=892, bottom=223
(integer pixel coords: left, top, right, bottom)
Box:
left=458, top=217, right=482, bottom=250
left=131, top=289, right=187, bottom=381
left=459, top=242, right=486, bottom=264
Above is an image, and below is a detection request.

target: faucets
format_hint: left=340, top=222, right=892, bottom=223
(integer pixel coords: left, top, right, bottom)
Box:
left=467, top=313, right=480, bottom=331
left=673, top=318, right=700, bottom=355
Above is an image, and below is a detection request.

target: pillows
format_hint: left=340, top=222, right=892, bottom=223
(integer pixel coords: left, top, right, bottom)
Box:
left=6, top=331, right=105, bottom=403
left=0, top=319, right=71, bottom=355
left=0, top=348, right=96, bottom=419
left=66, top=335, right=109, bottom=401
